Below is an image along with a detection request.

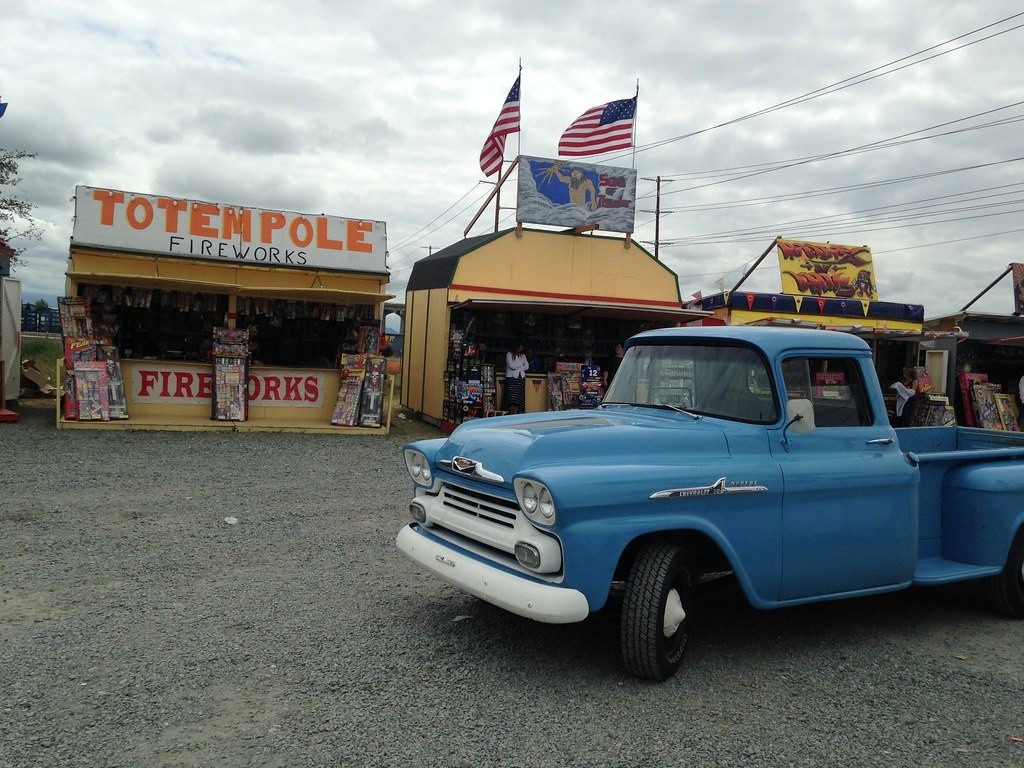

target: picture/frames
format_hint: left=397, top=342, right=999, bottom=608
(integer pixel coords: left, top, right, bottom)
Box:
left=993, top=393, right=1020, bottom=432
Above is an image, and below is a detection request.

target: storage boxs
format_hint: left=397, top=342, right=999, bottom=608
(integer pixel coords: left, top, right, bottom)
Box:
left=958, top=373, right=989, bottom=427
left=970, top=380, right=1003, bottom=430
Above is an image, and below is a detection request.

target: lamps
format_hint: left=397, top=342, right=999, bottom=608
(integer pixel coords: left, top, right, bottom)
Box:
left=791, top=319, right=800, bottom=324
left=852, top=324, right=862, bottom=329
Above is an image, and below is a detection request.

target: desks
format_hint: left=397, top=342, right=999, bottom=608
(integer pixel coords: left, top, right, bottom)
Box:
left=496, top=373, right=548, bottom=412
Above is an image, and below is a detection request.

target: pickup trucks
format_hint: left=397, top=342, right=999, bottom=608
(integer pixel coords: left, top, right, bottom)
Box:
left=394, top=325, right=1024, bottom=685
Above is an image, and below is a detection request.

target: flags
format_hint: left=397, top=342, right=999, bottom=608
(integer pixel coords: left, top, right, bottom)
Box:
left=558, top=96, right=637, bottom=156
left=480, top=76, right=520, bottom=178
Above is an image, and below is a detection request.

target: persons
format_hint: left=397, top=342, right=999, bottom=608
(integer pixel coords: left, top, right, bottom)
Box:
left=524, top=346, right=540, bottom=374
left=889, top=366, right=918, bottom=428
left=500, top=338, right=529, bottom=415
left=603, top=342, right=625, bottom=392
left=1017, top=375, right=1024, bottom=427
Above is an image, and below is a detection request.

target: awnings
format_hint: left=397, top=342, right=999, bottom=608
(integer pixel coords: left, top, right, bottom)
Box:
left=742, top=317, right=969, bottom=342
left=65, top=272, right=241, bottom=295
left=238, top=287, right=397, bottom=305
left=448, top=299, right=715, bottom=323
left=980, top=336, right=1024, bottom=347
